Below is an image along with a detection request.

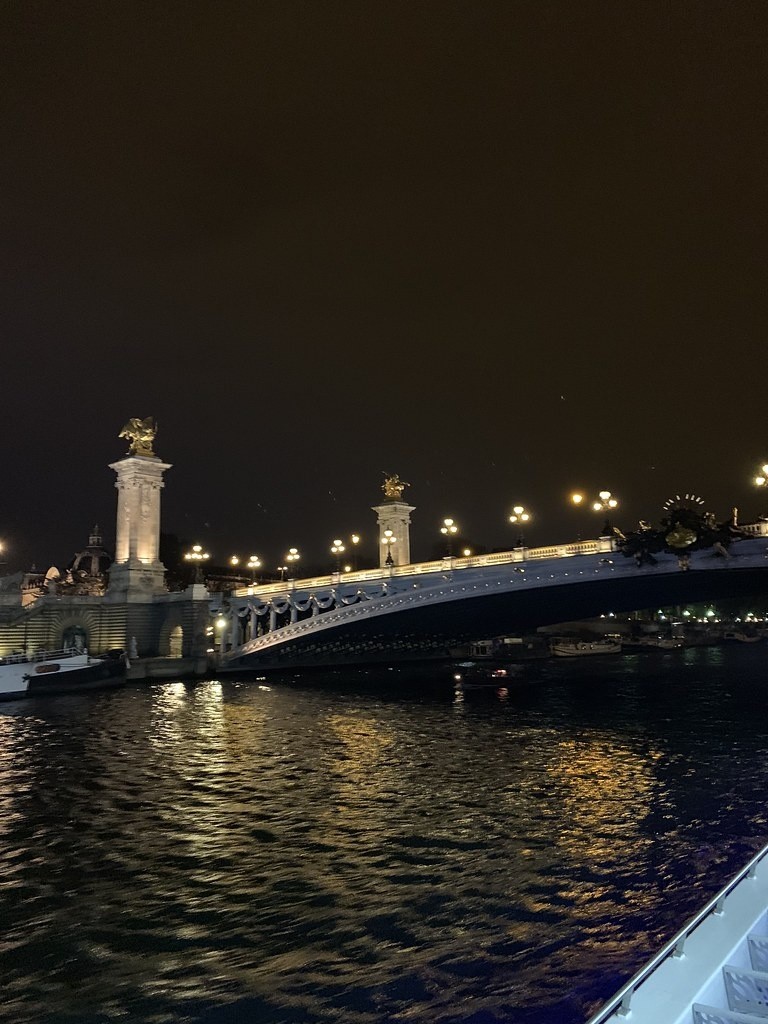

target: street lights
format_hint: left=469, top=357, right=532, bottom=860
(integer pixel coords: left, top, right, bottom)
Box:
left=593, top=490, right=618, bottom=536
left=286, top=547, right=301, bottom=581
left=247, top=555, right=260, bottom=585
left=439, top=519, right=457, bottom=559
left=183, top=545, right=212, bottom=584
left=331, top=539, right=345, bottom=575
left=277, top=567, right=288, bottom=581
left=509, top=505, right=530, bottom=551
left=352, top=535, right=360, bottom=571
left=381, top=526, right=397, bottom=568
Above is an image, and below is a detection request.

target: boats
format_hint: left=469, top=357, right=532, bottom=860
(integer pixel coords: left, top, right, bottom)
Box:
left=0, top=648, right=132, bottom=702
left=602, top=633, right=641, bottom=648
left=467, top=638, right=543, bottom=662
left=642, top=631, right=686, bottom=650
left=706, top=625, right=768, bottom=643
left=549, top=636, right=622, bottom=657
left=455, top=666, right=510, bottom=686
